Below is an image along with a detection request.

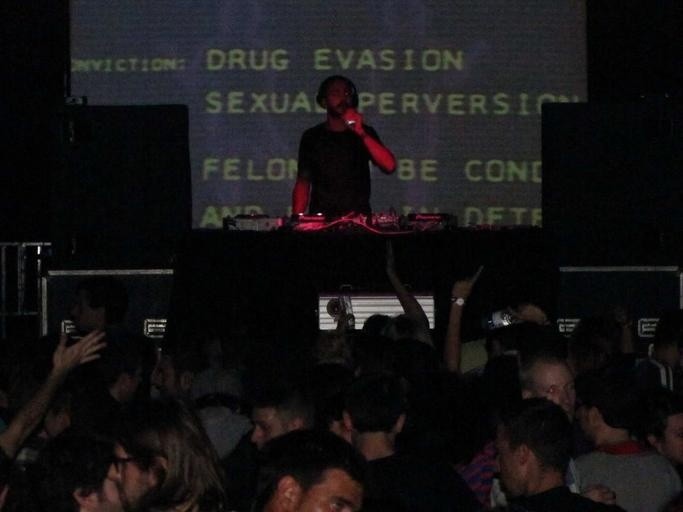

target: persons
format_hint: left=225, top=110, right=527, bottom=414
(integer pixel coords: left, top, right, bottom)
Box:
left=290, top=74, right=398, bottom=220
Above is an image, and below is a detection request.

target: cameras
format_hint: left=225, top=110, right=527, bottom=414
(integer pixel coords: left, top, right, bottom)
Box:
left=486, top=306, right=514, bottom=330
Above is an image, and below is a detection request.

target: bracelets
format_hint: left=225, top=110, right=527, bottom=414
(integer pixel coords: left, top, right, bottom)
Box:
left=361, top=130, right=368, bottom=140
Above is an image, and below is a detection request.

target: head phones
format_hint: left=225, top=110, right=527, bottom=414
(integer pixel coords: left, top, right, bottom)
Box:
left=318, top=74, right=361, bottom=109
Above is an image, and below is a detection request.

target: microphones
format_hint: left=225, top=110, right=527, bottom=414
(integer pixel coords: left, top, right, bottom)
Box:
left=405, top=211, right=443, bottom=223
